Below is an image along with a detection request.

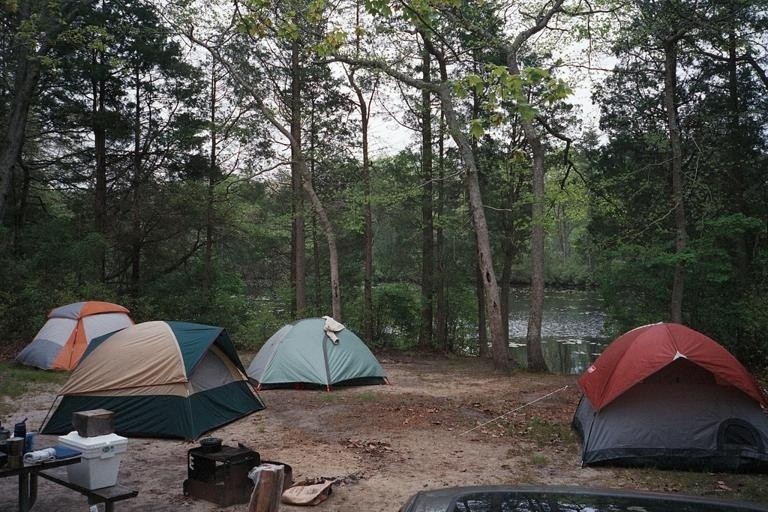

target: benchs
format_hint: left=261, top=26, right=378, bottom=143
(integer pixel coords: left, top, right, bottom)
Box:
left=39, top=465, right=140, bottom=511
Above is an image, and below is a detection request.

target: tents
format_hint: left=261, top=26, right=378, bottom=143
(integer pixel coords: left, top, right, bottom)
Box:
left=248, top=316, right=390, bottom=392
left=571, top=321, right=767, bottom=470
left=41, top=318, right=265, bottom=442
left=16, top=301, right=136, bottom=373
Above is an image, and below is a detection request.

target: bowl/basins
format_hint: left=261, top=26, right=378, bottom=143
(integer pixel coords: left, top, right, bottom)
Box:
left=200, top=438, right=223, bottom=452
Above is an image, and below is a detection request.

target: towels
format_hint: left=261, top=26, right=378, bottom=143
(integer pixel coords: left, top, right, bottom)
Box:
left=24, top=448, right=57, bottom=465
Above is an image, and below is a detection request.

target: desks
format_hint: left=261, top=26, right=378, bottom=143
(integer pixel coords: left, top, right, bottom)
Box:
left=1, top=447, right=82, bottom=511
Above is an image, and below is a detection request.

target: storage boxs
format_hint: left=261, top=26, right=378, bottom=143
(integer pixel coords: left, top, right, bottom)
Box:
left=57, top=430, right=129, bottom=493
left=72, top=406, right=115, bottom=437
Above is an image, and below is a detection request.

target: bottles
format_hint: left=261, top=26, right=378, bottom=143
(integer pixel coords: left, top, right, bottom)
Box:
left=0, top=422, right=27, bottom=471
